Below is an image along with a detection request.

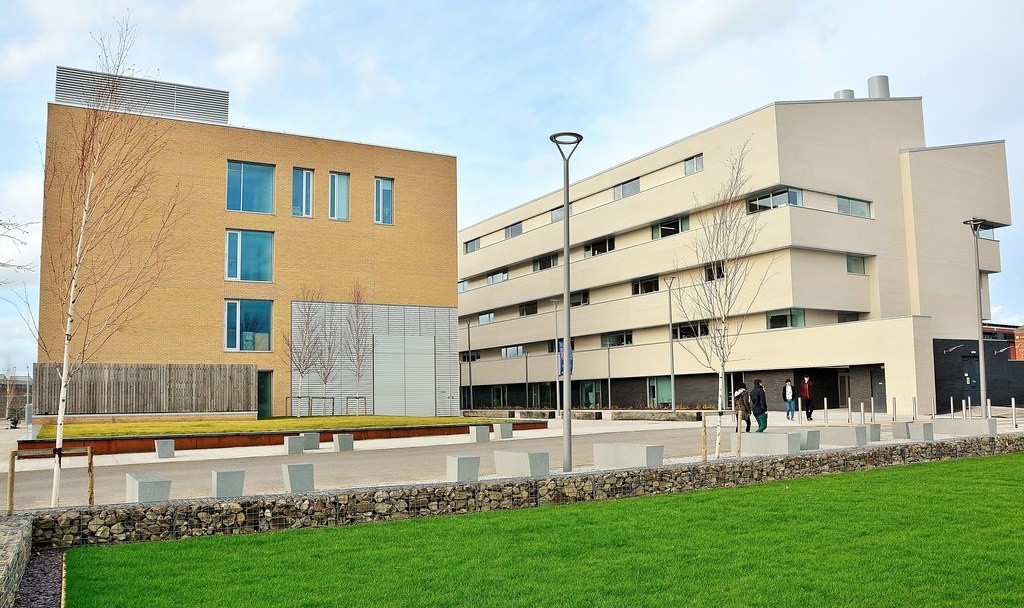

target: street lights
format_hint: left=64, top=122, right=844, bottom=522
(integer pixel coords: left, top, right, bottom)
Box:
left=548, top=132, right=586, bottom=464
left=549, top=299, right=561, bottom=415
left=662, top=275, right=676, bottom=412
left=463, top=317, right=473, bottom=409
left=963, top=217, right=986, bottom=418
left=604, top=342, right=613, bottom=410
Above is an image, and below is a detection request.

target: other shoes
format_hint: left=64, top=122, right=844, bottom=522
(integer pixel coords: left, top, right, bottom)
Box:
left=791, top=418, right=795, bottom=421
left=807, top=418, right=813, bottom=421
left=787, top=416, right=790, bottom=419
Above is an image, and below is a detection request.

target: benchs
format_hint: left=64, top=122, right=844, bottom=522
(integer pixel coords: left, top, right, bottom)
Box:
left=731, top=433, right=802, bottom=455
left=594, top=443, right=663, bottom=467
left=493, top=450, right=549, bottom=477
left=818, top=427, right=866, bottom=446
left=935, top=419, right=997, bottom=435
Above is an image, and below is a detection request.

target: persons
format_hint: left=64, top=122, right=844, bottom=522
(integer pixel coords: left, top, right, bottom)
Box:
left=782, top=379, right=796, bottom=420
left=800, top=376, right=814, bottom=421
left=750, top=378, right=768, bottom=433
left=735, top=382, right=751, bottom=433
